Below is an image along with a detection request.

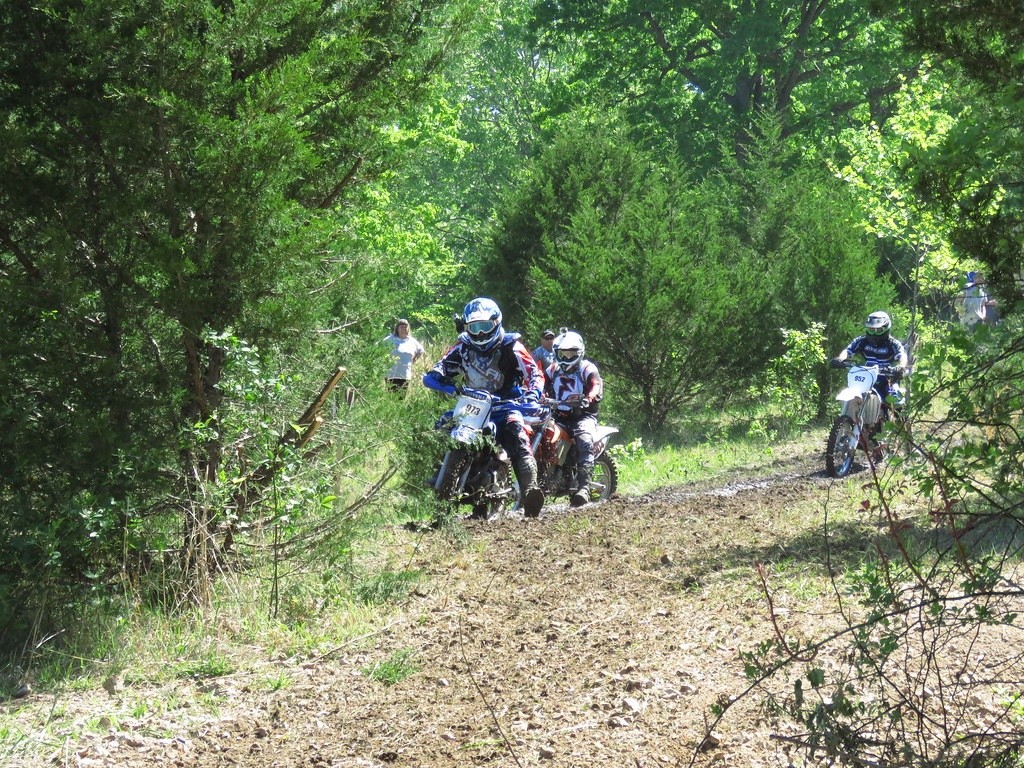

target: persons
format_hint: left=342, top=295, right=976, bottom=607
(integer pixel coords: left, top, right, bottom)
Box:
left=427, top=297, right=546, bottom=517
left=544, top=331, right=601, bottom=508
left=954, top=272, right=988, bottom=333
left=834, top=311, right=908, bottom=450
left=375, top=319, right=425, bottom=401
left=530, top=329, right=554, bottom=372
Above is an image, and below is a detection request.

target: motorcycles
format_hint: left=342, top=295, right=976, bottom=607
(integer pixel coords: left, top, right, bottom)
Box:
left=519, top=394, right=619, bottom=510
left=825, top=359, right=909, bottom=482
left=421, top=374, right=534, bottom=531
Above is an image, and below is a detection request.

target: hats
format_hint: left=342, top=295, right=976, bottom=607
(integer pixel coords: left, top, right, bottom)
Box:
left=541, top=329, right=555, bottom=339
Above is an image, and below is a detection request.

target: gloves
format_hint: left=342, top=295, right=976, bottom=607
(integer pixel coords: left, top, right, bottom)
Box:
left=892, top=365, right=905, bottom=380
left=830, top=357, right=845, bottom=369
left=427, top=371, right=442, bottom=381
left=580, top=399, right=590, bottom=409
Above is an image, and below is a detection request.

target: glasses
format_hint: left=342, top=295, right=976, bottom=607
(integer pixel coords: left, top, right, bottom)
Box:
left=467, top=320, right=497, bottom=335
left=865, top=326, right=886, bottom=336
left=557, top=349, right=576, bottom=360
left=544, top=335, right=554, bottom=340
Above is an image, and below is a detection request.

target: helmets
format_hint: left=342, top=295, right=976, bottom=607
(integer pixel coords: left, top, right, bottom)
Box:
left=552, top=327, right=585, bottom=372
left=865, top=311, right=891, bottom=347
left=461, top=297, right=502, bottom=351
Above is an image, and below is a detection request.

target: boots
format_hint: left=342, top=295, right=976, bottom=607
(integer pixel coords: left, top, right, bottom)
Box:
left=426, top=471, right=453, bottom=487
left=512, top=456, right=544, bottom=517
left=570, top=464, right=594, bottom=507
